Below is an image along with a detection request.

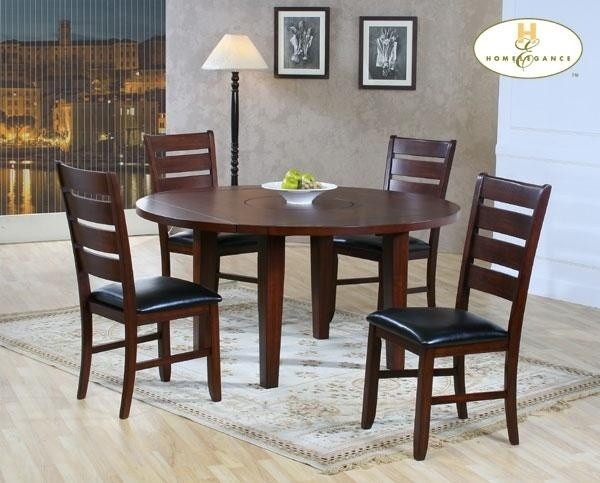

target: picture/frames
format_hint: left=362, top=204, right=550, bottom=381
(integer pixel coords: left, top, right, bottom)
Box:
left=359, top=16, right=417, bottom=90
left=274, top=6, right=330, bottom=79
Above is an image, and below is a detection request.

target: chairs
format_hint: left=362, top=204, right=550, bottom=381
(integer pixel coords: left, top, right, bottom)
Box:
left=329, top=136, right=457, bottom=323
left=361, top=173, right=552, bottom=461
left=55, top=159, right=223, bottom=419
left=142, top=129, right=259, bottom=292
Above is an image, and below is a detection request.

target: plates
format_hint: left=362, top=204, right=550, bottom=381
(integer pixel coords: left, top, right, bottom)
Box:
left=260, top=180, right=339, bottom=205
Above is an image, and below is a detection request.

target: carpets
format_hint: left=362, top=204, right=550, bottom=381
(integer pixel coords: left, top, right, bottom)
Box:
left=0, top=278, right=600, bottom=476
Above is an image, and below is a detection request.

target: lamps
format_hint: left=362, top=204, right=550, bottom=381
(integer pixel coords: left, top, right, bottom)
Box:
left=200, top=34, right=268, bottom=187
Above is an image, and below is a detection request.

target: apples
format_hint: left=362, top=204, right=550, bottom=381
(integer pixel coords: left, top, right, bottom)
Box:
left=281, top=168, right=314, bottom=189
